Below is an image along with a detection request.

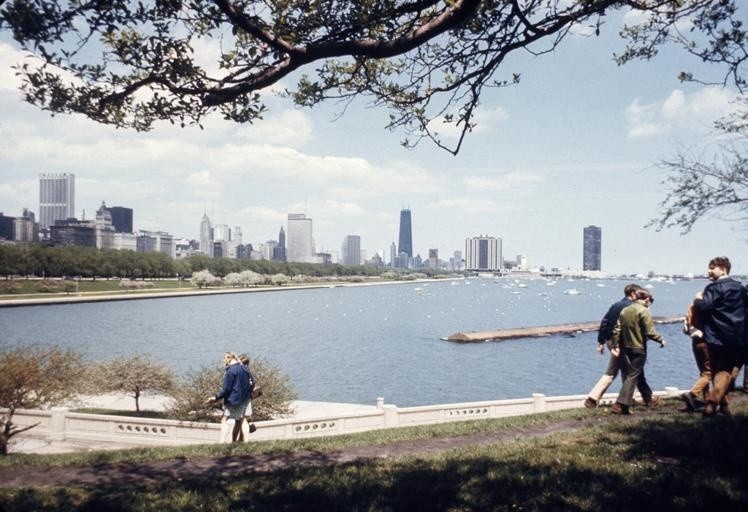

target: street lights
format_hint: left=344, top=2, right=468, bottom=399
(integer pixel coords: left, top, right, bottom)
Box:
left=176, top=273, right=179, bottom=288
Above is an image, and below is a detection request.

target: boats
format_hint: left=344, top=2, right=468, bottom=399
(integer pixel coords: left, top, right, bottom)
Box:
left=413, top=276, right=654, bottom=298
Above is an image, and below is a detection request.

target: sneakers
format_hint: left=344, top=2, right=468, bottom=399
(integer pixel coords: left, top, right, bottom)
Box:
left=683, top=392, right=731, bottom=415
left=612, top=402, right=634, bottom=415
left=584, top=398, right=596, bottom=409
left=647, top=396, right=660, bottom=408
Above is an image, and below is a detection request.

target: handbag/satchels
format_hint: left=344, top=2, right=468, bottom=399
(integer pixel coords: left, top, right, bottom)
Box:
left=250, top=386, right=264, bottom=400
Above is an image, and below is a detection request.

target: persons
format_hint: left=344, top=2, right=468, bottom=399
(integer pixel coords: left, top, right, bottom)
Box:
left=582, top=283, right=643, bottom=409
left=202, top=350, right=255, bottom=444
left=218, top=354, right=250, bottom=444
left=609, top=289, right=666, bottom=414
left=680, top=290, right=713, bottom=412
left=694, top=255, right=748, bottom=427
